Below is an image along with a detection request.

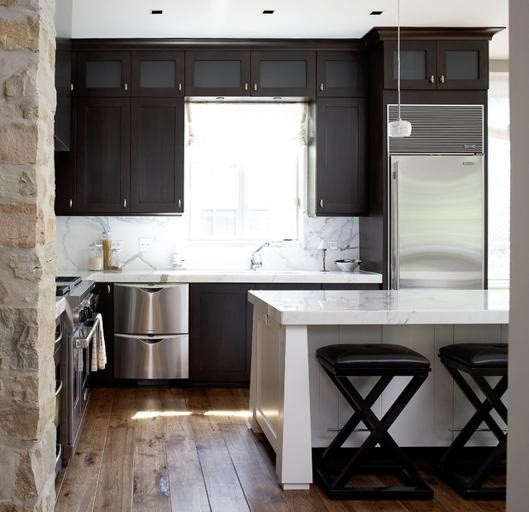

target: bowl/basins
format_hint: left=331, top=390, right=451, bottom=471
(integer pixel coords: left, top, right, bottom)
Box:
left=335, top=259, right=362, bottom=271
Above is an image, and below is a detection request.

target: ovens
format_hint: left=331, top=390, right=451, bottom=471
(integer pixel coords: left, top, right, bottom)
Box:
left=64, top=291, right=105, bottom=459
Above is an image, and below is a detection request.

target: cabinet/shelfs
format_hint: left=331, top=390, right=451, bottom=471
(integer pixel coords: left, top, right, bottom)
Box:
left=186, top=49, right=316, bottom=96
left=70, top=50, right=184, bottom=98
left=317, top=51, right=371, bottom=98
left=189, top=283, right=381, bottom=388
left=363, top=41, right=489, bottom=90
left=75, top=96, right=184, bottom=212
left=318, top=99, right=383, bottom=216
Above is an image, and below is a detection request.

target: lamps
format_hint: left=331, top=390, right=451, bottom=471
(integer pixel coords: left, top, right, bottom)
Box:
left=387, top=0, right=412, bottom=137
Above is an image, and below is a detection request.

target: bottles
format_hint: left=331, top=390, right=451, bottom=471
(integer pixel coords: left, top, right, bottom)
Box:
left=88, top=231, right=122, bottom=271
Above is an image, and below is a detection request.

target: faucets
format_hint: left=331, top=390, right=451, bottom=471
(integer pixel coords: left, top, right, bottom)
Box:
left=249, top=240, right=272, bottom=271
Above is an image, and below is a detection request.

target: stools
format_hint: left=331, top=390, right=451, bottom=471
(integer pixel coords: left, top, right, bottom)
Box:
left=437, top=343, right=507, bottom=501
left=315, top=343, right=435, bottom=501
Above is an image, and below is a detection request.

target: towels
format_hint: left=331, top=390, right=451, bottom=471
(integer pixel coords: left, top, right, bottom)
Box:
left=90, top=314, right=108, bottom=372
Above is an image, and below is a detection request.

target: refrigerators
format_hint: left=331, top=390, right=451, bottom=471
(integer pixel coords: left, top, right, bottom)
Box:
left=390, top=155, right=485, bottom=289
